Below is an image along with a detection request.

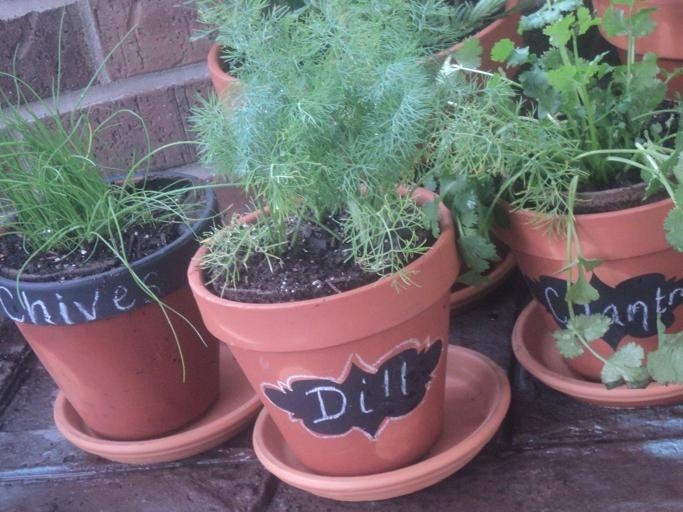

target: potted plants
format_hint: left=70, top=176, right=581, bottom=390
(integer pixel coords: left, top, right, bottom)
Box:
left=2, top=0, right=681, bottom=501
